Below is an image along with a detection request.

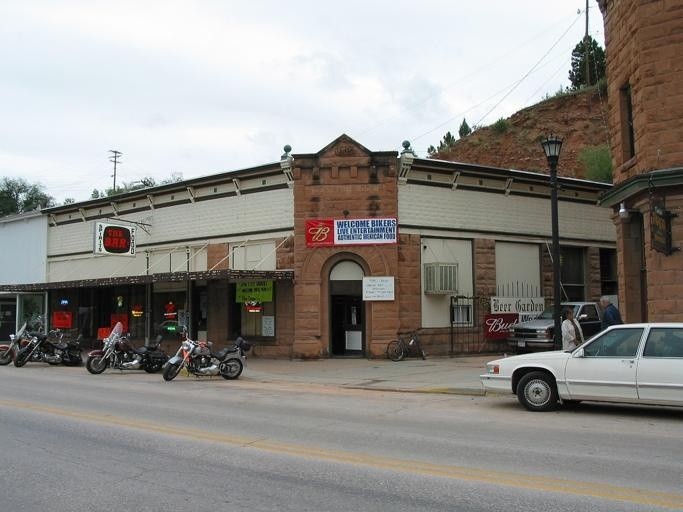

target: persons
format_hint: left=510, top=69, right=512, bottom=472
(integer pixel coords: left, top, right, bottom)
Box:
left=559, top=306, right=584, bottom=349
left=599, top=296, right=623, bottom=331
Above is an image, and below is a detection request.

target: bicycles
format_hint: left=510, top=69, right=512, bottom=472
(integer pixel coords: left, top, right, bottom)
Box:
left=387, top=328, right=426, bottom=361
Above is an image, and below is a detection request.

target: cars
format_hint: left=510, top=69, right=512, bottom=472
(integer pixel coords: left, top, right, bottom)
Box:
left=507, top=301, right=619, bottom=353
left=479, top=323, right=683, bottom=412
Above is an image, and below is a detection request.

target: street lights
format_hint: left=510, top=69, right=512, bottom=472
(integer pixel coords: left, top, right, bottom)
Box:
left=538, top=132, right=565, bottom=349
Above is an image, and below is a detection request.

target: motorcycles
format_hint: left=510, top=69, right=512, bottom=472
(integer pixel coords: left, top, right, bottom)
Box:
left=87, top=321, right=170, bottom=374
left=162, top=325, right=251, bottom=380
left=0, top=320, right=83, bottom=367
